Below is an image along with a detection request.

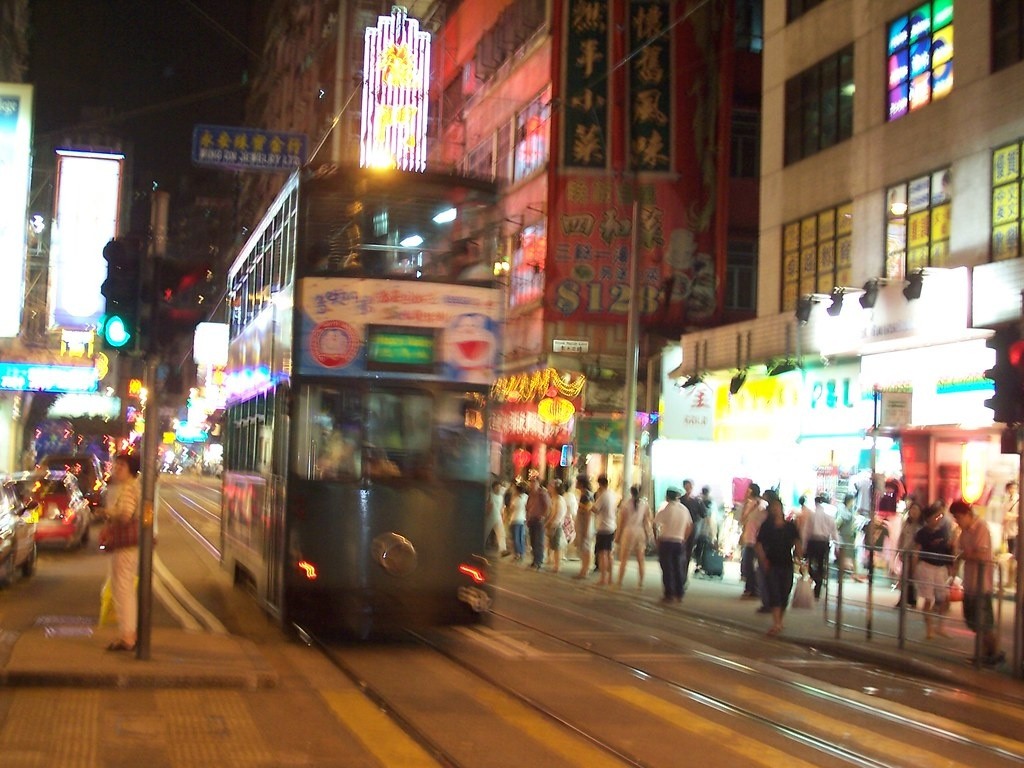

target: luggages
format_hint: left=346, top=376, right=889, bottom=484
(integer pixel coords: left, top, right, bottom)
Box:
left=703, top=540, right=724, bottom=577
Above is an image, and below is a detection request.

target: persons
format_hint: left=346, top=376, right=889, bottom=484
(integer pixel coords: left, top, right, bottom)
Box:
left=490, top=470, right=1019, bottom=668
left=94, top=454, right=143, bottom=650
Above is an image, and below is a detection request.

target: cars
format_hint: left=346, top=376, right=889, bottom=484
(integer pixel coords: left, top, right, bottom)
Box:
left=12, top=467, right=92, bottom=553
left=1, top=472, right=42, bottom=589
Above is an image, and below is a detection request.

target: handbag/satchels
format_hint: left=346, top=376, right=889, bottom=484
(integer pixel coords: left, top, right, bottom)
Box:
left=96, top=497, right=140, bottom=552
left=562, top=514, right=576, bottom=543
left=791, top=569, right=815, bottom=609
left=644, top=518, right=653, bottom=543
left=96, top=573, right=139, bottom=630
left=948, top=576, right=964, bottom=602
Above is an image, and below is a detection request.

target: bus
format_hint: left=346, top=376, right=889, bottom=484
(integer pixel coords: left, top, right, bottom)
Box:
left=220, top=158, right=508, bottom=641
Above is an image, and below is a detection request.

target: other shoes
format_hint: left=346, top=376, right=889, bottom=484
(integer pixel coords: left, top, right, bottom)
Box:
left=767, top=624, right=784, bottom=635
left=741, top=590, right=759, bottom=599
left=104, top=634, right=138, bottom=650
left=965, top=649, right=1006, bottom=671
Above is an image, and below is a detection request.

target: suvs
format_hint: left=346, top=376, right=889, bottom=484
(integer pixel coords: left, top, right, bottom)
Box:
left=33, top=452, right=109, bottom=512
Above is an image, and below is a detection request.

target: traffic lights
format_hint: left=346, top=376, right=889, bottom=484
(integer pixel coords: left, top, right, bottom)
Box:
left=979, top=311, right=1023, bottom=424
left=98, top=227, right=146, bottom=356
left=149, top=256, right=189, bottom=341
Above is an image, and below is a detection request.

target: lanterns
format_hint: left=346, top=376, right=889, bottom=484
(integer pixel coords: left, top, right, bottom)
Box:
left=962, top=442, right=984, bottom=502
left=538, top=397, right=575, bottom=445
left=511, top=444, right=561, bottom=473
